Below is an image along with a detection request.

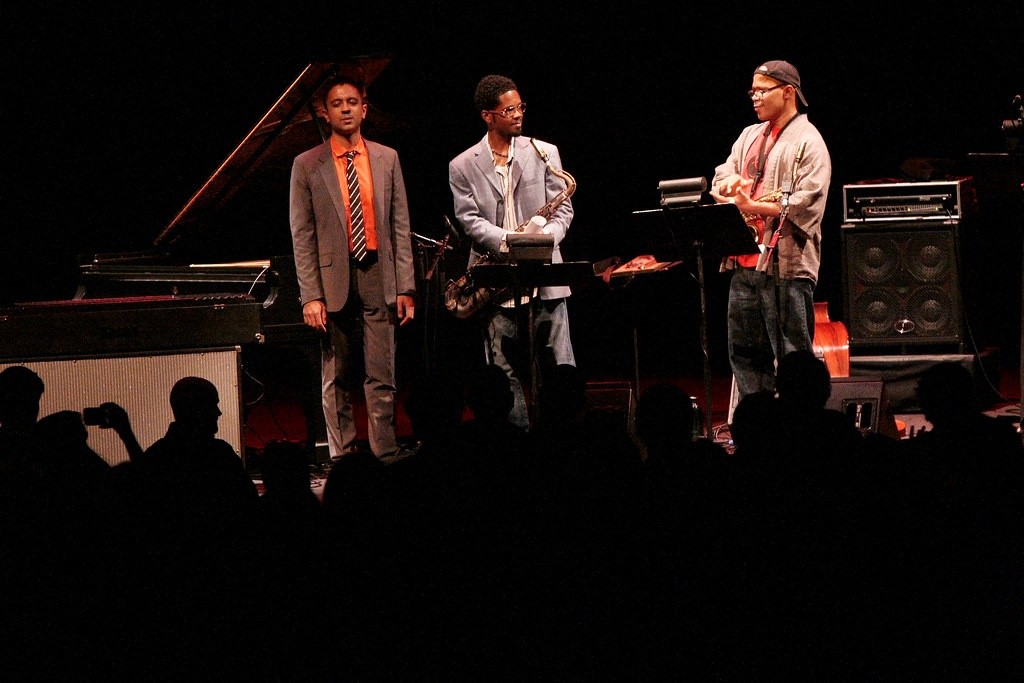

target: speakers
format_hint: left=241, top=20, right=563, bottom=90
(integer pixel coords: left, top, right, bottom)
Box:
left=837, top=219, right=968, bottom=350
left=585, top=381, right=648, bottom=462
left=0, top=345, right=244, bottom=465
left=821, top=376, right=901, bottom=443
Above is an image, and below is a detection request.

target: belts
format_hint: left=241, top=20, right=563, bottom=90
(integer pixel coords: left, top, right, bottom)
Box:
left=348, top=255, right=379, bottom=263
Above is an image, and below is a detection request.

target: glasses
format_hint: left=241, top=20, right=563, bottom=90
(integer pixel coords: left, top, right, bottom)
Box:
left=748, top=83, right=788, bottom=99
left=488, top=103, right=527, bottom=117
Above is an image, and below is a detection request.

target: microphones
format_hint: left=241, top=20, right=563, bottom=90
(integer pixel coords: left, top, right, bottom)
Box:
left=443, top=214, right=459, bottom=240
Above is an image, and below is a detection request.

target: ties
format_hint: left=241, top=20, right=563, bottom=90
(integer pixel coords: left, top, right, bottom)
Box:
left=345, top=150, right=367, bottom=262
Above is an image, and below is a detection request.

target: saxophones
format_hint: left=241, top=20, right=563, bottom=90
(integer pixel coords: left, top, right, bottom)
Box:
left=444, top=138, right=576, bottom=318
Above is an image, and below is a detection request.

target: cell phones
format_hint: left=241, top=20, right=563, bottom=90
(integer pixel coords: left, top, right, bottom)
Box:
left=84, top=407, right=110, bottom=426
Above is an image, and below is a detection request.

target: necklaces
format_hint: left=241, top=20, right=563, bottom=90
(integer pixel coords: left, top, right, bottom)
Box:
left=491, top=150, right=509, bottom=157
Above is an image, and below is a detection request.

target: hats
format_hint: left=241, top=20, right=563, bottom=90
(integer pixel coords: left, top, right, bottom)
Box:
left=754, top=60, right=808, bottom=106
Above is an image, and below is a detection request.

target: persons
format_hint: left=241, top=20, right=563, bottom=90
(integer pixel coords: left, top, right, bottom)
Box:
left=1, top=352, right=1024, bottom=683
left=288, top=76, right=416, bottom=461
left=448, top=75, right=577, bottom=427
left=709, top=60, right=832, bottom=398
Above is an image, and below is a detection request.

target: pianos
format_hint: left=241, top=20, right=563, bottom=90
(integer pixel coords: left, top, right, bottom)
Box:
left=80, top=254, right=326, bottom=441
